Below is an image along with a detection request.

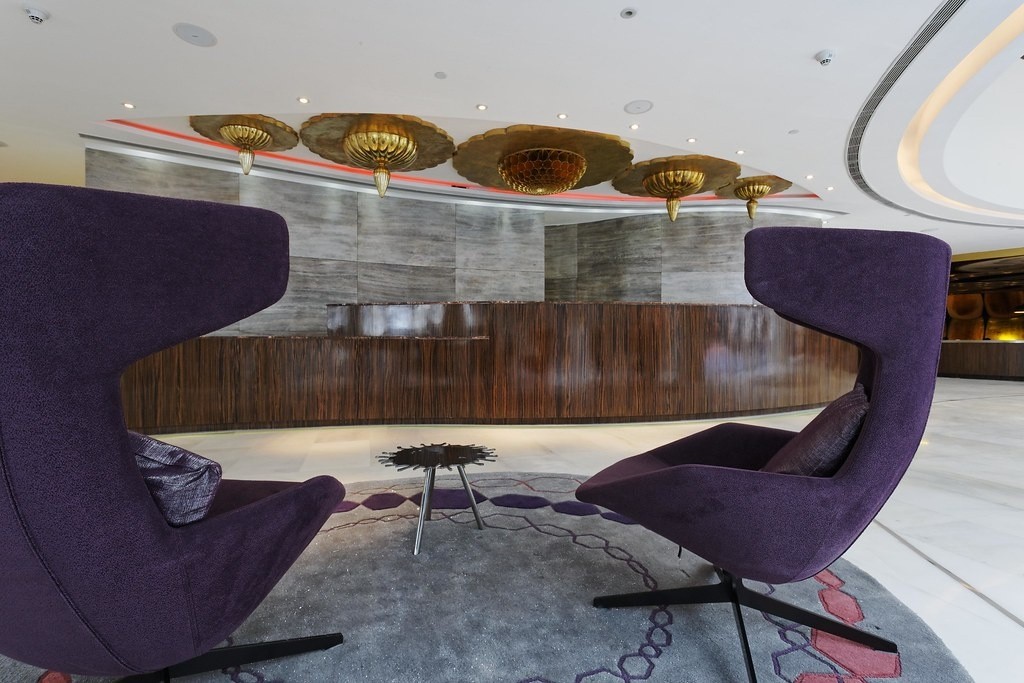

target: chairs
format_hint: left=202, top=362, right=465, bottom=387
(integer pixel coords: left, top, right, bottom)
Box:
left=0, top=181, right=343, bottom=683
left=574, top=228, right=954, bottom=683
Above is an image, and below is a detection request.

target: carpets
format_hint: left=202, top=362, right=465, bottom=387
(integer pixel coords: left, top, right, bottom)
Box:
left=1, top=464, right=980, bottom=683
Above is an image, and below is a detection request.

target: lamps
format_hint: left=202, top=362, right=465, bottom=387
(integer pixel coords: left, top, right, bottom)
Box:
left=186, top=113, right=792, bottom=221
